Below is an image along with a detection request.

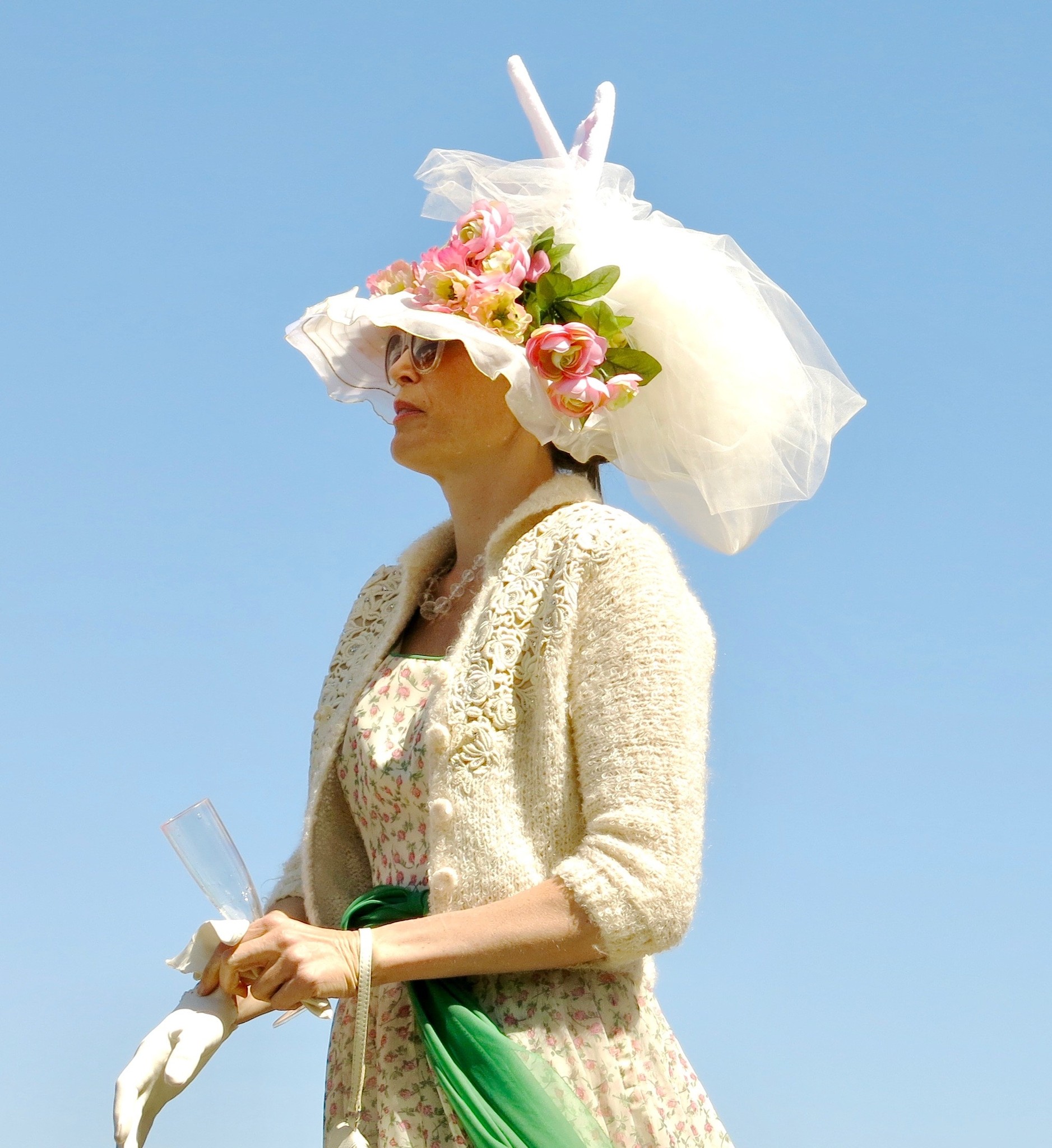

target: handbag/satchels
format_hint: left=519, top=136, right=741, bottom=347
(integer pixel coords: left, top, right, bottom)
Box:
left=326, top=927, right=374, bottom=1148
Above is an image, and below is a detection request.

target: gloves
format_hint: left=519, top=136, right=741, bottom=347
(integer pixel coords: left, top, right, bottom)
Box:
left=165, top=920, right=333, bottom=1028
left=114, top=986, right=238, bottom=1148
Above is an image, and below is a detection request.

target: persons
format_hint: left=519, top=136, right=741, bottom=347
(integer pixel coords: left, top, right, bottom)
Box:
left=112, top=261, right=736, bottom=1147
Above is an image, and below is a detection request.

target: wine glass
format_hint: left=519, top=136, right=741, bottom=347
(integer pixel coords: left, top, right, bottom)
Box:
left=161, top=798, right=307, bottom=1028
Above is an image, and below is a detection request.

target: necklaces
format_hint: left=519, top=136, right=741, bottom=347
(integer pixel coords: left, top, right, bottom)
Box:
left=416, top=549, right=486, bottom=623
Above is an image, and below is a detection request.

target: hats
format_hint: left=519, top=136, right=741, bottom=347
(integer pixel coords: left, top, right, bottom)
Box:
left=284, top=54, right=869, bottom=556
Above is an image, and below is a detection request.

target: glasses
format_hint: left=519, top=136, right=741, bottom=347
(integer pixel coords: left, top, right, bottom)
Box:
left=384, top=331, right=445, bottom=388
